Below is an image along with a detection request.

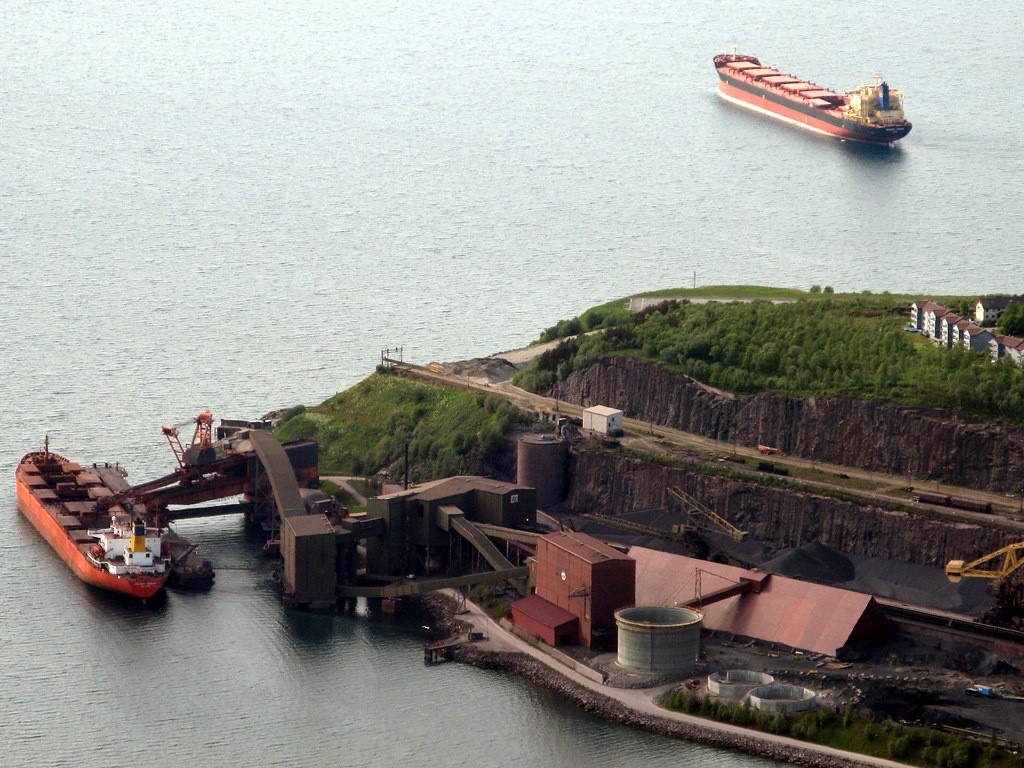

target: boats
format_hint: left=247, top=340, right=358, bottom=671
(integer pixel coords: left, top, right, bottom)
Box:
left=712, top=46, right=913, bottom=143
left=14, top=433, right=177, bottom=601
